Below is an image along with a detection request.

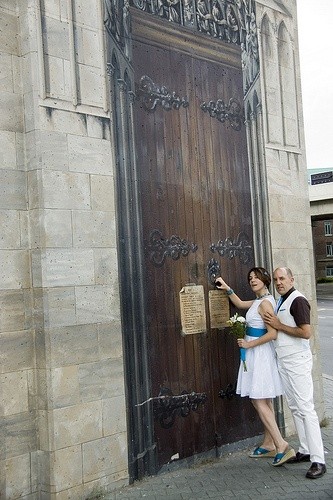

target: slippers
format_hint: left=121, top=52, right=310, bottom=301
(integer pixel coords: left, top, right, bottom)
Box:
left=249, top=446, right=276, bottom=458
left=272, top=449, right=296, bottom=467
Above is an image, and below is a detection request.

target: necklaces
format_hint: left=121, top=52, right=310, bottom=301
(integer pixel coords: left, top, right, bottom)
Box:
left=256, top=293, right=271, bottom=300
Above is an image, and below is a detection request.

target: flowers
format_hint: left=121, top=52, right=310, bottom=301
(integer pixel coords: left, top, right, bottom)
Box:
left=226, top=311, right=250, bottom=371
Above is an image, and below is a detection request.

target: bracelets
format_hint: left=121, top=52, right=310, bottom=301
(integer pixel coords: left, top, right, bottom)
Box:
left=227, top=289, right=233, bottom=296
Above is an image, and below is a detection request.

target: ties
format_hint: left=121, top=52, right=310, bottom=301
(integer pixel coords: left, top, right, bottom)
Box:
left=276, top=297, right=283, bottom=313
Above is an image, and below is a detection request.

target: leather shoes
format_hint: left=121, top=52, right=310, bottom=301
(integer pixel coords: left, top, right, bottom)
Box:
left=306, top=462, right=326, bottom=479
left=286, top=452, right=310, bottom=464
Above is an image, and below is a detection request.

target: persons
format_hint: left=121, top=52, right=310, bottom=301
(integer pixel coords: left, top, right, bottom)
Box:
left=262, top=267, right=327, bottom=479
left=215, top=267, right=296, bottom=467
